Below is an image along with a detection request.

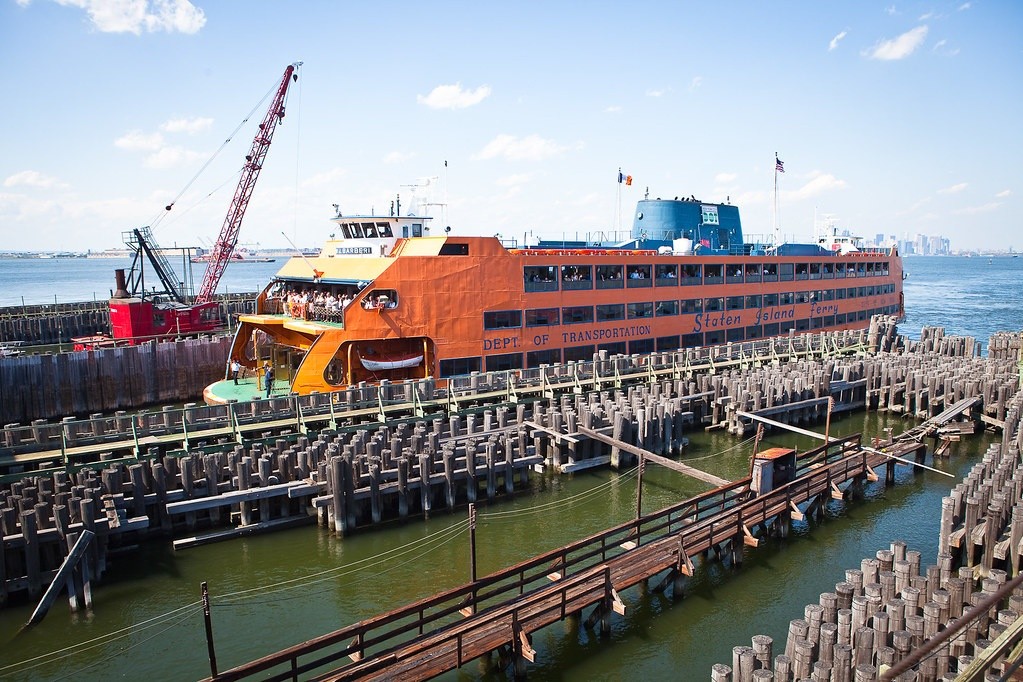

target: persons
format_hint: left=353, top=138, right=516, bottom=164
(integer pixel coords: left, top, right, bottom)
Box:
left=763, top=267, right=774, bottom=275
left=281, top=289, right=396, bottom=324
left=728, top=267, right=742, bottom=276
left=629, top=268, right=646, bottom=279
left=525, top=271, right=554, bottom=283
left=564, top=271, right=584, bottom=281
left=746, top=268, right=760, bottom=276
left=661, top=267, right=677, bottom=279
left=231, top=359, right=241, bottom=385
left=263, top=365, right=275, bottom=398
left=600, top=271, right=622, bottom=282
left=824, top=264, right=865, bottom=273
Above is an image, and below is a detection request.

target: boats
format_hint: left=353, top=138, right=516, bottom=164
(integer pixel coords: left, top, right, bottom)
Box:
left=0, top=341, right=28, bottom=359
left=189, top=235, right=275, bottom=262
left=202, top=151, right=906, bottom=407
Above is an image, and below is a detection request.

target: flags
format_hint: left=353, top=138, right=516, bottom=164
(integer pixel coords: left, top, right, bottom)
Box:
left=618, top=174, right=631, bottom=185
left=776, top=159, right=784, bottom=173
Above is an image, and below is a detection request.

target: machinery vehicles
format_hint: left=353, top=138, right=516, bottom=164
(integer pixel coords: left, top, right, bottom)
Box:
left=70, top=60, right=303, bottom=351
